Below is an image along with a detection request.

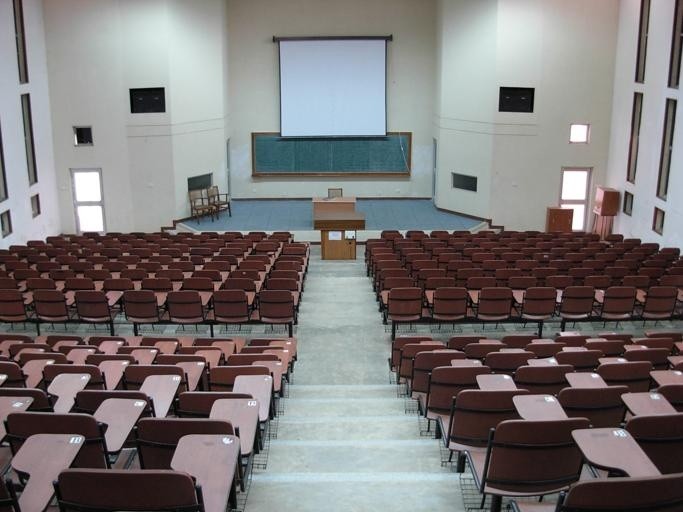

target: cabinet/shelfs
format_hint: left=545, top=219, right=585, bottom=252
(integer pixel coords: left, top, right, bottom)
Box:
left=545, top=207, right=573, bottom=232
left=592, top=187, right=620, bottom=216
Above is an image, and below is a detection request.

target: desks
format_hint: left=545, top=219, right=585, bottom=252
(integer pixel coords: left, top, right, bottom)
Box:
left=312, top=195, right=356, bottom=216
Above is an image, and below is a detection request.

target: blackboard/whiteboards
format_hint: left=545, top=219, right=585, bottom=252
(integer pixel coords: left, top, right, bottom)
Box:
left=250, top=132, right=412, bottom=178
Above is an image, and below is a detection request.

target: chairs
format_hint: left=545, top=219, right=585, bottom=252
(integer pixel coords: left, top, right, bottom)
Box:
left=328, top=188, right=342, bottom=197
left=189, top=187, right=213, bottom=225
left=206, top=186, right=231, bottom=220
